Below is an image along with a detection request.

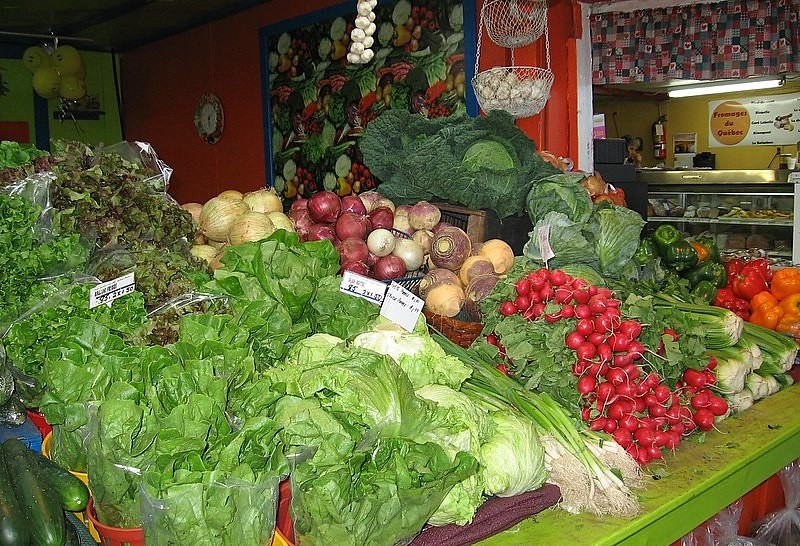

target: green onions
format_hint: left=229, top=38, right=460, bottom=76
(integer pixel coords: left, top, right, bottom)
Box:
left=427, top=327, right=648, bottom=519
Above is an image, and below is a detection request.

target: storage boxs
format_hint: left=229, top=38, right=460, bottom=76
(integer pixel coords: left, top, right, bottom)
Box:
left=594, top=138, right=648, bottom=220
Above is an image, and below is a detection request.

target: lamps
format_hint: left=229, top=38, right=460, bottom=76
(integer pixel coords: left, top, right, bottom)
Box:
left=668, top=77, right=784, bottom=96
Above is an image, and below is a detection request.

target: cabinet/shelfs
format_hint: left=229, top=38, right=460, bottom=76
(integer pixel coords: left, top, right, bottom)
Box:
left=649, top=190, right=796, bottom=258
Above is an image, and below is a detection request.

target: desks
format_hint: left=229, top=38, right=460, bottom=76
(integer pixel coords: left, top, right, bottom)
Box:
left=476, top=381, right=800, bottom=546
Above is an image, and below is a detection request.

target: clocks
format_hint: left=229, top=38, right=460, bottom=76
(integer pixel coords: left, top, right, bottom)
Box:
left=192, top=94, right=223, bottom=145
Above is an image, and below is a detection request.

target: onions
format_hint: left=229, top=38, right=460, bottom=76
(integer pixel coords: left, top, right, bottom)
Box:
left=180, top=186, right=425, bottom=281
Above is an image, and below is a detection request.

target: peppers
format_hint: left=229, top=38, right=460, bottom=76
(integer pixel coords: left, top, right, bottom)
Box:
left=633, top=225, right=800, bottom=344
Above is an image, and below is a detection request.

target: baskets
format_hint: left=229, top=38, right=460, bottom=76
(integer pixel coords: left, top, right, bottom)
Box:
left=470, top=66, right=558, bottom=121
left=479, top=1, right=550, bottom=49
left=421, top=308, right=485, bottom=349
left=378, top=226, right=431, bottom=291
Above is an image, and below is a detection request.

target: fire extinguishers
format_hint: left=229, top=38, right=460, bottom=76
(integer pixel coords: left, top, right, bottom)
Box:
left=652, top=114, right=668, bottom=160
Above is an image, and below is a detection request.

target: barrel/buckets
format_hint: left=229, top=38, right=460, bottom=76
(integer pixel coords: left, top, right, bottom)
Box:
left=270, top=526, right=295, bottom=546
left=86, top=497, right=145, bottom=546
left=27, top=408, right=53, bottom=440
left=40, top=430, right=104, bottom=544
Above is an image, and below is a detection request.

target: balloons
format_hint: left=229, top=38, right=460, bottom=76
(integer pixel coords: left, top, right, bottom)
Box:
left=23, top=45, right=87, bottom=100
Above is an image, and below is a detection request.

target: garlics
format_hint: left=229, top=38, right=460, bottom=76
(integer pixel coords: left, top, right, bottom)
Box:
left=474, top=66, right=548, bottom=120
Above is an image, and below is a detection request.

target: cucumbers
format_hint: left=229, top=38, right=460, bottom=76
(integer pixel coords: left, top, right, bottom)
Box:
left=0, top=366, right=91, bottom=546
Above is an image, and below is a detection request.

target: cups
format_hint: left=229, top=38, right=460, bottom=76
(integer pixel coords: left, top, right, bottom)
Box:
left=787, top=159, right=796, bottom=170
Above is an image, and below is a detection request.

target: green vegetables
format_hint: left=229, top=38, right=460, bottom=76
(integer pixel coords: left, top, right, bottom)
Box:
left=286, top=435, right=482, bottom=546
left=0, top=140, right=227, bottom=407
left=38, top=230, right=382, bottom=546
left=603, top=277, right=799, bottom=411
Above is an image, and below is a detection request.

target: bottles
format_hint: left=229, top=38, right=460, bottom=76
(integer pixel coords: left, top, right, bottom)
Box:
left=778, top=154, right=792, bottom=170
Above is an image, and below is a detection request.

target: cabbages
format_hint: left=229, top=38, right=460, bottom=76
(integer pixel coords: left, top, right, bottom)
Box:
left=268, top=311, right=547, bottom=527
left=360, top=108, right=645, bottom=287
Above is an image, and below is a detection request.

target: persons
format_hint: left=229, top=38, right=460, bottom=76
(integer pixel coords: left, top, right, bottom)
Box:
left=621, top=135, right=642, bottom=167
